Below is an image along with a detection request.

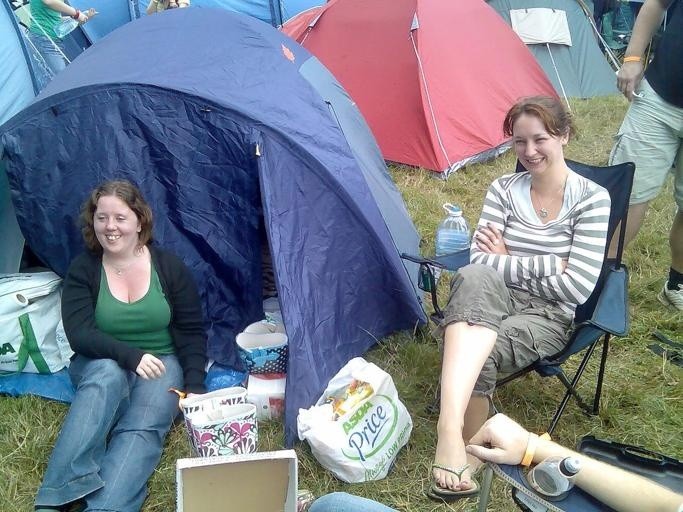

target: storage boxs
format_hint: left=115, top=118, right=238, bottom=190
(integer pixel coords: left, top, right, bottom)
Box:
left=248, top=374, right=285, bottom=418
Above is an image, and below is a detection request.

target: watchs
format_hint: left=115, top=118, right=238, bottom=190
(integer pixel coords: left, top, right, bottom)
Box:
left=71, top=9, right=80, bottom=20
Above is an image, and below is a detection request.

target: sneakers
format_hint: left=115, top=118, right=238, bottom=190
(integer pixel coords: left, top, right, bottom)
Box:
left=657, top=281, right=683, bottom=312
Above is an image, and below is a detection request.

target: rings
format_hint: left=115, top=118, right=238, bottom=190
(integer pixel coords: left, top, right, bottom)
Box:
left=493, top=239, right=499, bottom=246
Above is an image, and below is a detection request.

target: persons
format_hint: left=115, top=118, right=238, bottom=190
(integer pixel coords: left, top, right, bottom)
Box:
left=306, top=413, right=683, bottom=511
left=35, top=182, right=208, bottom=512
left=28, top=0, right=95, bottom=77
left=608, top=0, right=683, bottom=314
left=595, top=0, right=628, bottom=49
left=145, top=0, right=190, bottom=15
left=429, top=96, right=611, bottom=498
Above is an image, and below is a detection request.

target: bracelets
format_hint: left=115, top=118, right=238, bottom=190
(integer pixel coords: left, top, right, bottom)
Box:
left=521, top=431, right=552, bottom=467
left=167, top=1, right=176, bottom=4
left=622, top=57, right=641, bottom=63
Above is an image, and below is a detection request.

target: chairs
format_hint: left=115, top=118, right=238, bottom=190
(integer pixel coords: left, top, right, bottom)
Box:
left=479, top=461, right=611, bottom=512
left=602, top=12, right=629, bottom=67
left=400, top=157, right=635, bottom=436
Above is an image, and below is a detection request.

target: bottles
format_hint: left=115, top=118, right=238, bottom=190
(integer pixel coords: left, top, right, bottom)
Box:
left=511, top=456, right=582, bottom=512
left=435, top=203, right=471, bottom=256
left=53, top=10, right=96, bottom=38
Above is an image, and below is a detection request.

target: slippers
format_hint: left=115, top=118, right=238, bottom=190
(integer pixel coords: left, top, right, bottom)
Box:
left=428, top=464, right=480, bottom=502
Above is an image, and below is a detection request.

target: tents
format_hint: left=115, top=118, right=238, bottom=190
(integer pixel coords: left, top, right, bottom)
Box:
left=0, top=1, right=55, bottom=276
left=486, top=0, right=622, bottom=97
left=279, top=0, right=561, bottom=180
left=65, top=0, right=330, bottom=64
left=0, top=8, right=429, bottom=451
